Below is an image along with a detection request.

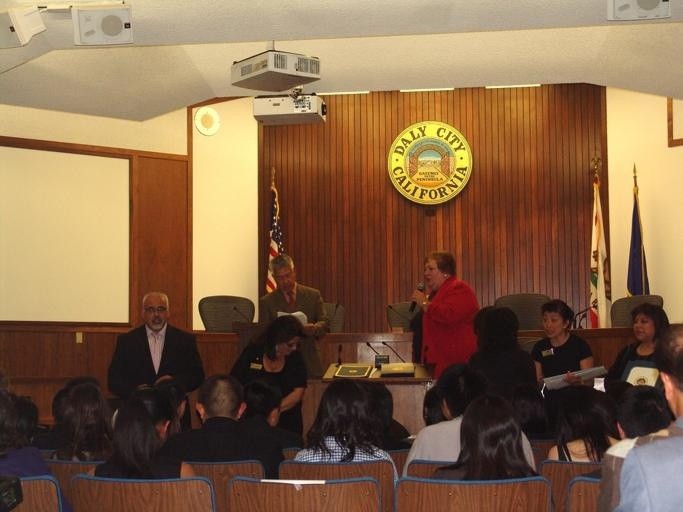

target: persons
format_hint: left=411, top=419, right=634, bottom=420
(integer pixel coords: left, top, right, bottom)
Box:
left=231, top=384, right=275, bottom=478
left=294, top=380, right=399, bottom=510
left=364, top=382, right=411, bottom=453
left=611, top=323, right=683, bottom=512
left=259, top=254, right=329, bottom=381
left=154, top=375, right=279, bottom=480
left=470, top=304, right=533, bottom=390
left=500, top=387, right=547, bottom=440
left=227, top=317, right=309, bottom=452
left=0, top=385, right=176, bottom=512
left=606, top=386, right=674, bottom=462
left=401, top=362, right=538, bottom=476
left=105, top=291, right=203, bottom=408
left=605, top=306, right=673, bottom=391
left=545, top=386, right=622, bottom=463
left=410, top=252, right=479, bottom=380
left=428, top=395, right=541, bottom=481
left=529, top=300, right=597, bottom=389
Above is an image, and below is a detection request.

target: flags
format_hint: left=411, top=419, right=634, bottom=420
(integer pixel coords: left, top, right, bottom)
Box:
left=265, top=186, right=286, bottom=296
left=626, top=183, right=651, bottom=298
left=588, top=175, right=608, bottom=330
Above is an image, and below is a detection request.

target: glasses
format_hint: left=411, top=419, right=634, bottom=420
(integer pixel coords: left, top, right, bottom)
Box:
left=145, top=305, right=168, bottom=313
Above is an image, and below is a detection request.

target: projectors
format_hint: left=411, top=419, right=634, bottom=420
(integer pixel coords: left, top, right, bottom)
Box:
left=253, top=92, right=328, bottom=124
left=229, top=50, right=322, bottom=91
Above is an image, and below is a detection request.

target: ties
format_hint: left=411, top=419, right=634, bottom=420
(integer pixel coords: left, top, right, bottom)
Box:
left=151, top=332, right=160, bottom=372
left=285, top=290, right=296, bottom=311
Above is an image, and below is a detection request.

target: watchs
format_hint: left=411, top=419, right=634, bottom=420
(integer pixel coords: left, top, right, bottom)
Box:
left=421, top=301, right=428, bottom=310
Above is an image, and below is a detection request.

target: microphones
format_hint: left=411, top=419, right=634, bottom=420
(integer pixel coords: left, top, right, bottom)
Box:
left=573, top=305, right=597, bottom=328
left=366, top=342, right=380, bottom=356
left=578, top=313, right=586, bottom=327
left=233, top=306, right=251, bottom=322
left=409, top=282, right=426, bottom=313
left=382, top=340, right=406, bottom=363
left=388, top=304, right=411, bottom=322
left=337, top=345, right=341, bottom=364
left=330, top=301, right=339, bottom=321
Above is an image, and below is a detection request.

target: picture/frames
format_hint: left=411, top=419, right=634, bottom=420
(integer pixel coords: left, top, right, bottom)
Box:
left=666, top=97, right=683, bottom=148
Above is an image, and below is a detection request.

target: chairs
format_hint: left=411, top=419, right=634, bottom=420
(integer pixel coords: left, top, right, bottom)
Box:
left=611, top=296, right=664, bottom=329
left=326, top=304, right=346, bottom=334
left=281, top=458, right=393, bottom=511
left=45, top=462, right=104, bottom=488
left=198, top=296, right=255, bottom=332
left=282, top=446, right=302, bottom=457
left=387, top=301, right=422, bottom=330
left=542, top=458, right=604, bottom=480
left=66, top=475, right=215, bottom=512
left=495, top=294, right=553, bottom=330
left=531, top=439, right=555, bottom=465
left=384, top=449, right=410, bottom=474
left=225, top=476, right=380, bottom=512
left=409, top=461, right=459, bottom=482
left=560, top=476, right=604, bottom=512
left=18, top=476, right=62, bottom=511
left=185, top=458, right=263, bottom=503
left=393, top=475, right=553, bottom=512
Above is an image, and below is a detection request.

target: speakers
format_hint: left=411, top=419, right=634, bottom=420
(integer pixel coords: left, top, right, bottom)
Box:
left=0, top=5, right=46, bottom=50
left=605, top=0, right=672, bottom=22
left=71, top=5, right=134, bottom=47
